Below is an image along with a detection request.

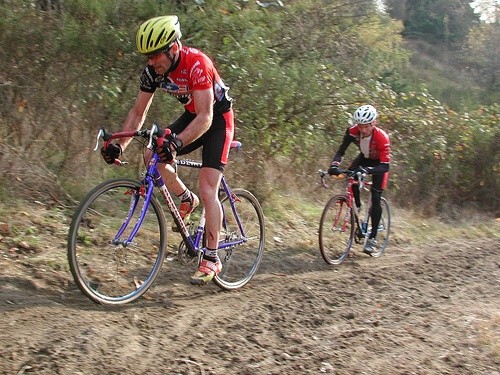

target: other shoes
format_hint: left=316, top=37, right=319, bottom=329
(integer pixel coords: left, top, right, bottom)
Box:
left=358, top=201, right=363, bottom=211
left=363, top=238, right=378, bottom=253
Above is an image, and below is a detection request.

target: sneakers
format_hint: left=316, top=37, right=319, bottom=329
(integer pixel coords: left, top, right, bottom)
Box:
left=172, top=191, right=200, bottom=232
left=189, top=256, right=222, bottom=285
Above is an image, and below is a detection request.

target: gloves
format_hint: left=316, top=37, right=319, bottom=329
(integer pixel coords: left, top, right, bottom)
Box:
left=328, top=165, right=340, bottom=176
left=364, top=167, right=375, bottom=174
left=156, top=139, right=183, bottom=164
left=101, top=143, right=122, bottom=165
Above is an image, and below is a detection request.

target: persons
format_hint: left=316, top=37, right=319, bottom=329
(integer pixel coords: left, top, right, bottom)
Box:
left=328, top=105, right=391, bottom=253
left=101, top=15, right=233, bottom=284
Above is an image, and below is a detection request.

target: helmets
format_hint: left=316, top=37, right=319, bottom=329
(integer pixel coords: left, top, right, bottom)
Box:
left=135, top=15, right=182, bottom=53
left=353, top=105, right=376, bottom=124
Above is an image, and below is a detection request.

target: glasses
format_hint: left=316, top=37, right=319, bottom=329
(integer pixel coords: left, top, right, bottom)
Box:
left=147, top=43, right=174, bottom=60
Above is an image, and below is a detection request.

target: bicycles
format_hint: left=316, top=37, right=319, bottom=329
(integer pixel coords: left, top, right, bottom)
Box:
left=66, top=123, right=265, bottom=307
left=317, top=166, right=393, bottom=266
